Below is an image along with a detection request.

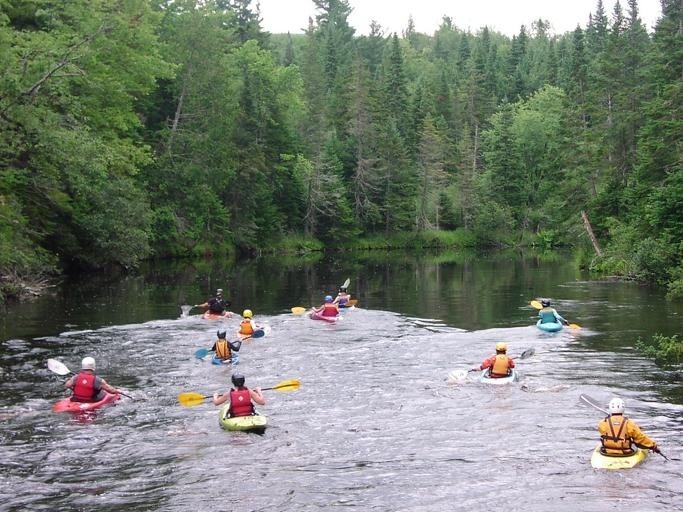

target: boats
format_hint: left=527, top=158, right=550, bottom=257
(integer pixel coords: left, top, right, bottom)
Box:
left=218, top=402, right=268, bottom=432
left=237, top=323, right=271, bottom=340
left=536, top=318, right=562, bottom=334
left=52, top=386, right=120, bottom=413
left=201, top=310, right=232, bottom=322
left=590, top=439, right=648, bottom=469
left=481, top=365, right=518, bottom=387
left=211, top=351, right=240, bottom=365
left=306, top=309, right=344, bottom=324
left=336, top=298, right=356, bottom=309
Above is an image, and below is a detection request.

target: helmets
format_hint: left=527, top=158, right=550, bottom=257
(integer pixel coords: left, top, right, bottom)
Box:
left=541, top=298, right=550, bottom=308
left=217, top=330, right=227, bottom=339
left=231, top=371, right=246, bottom=387
left=338, top=286, right=348, bottom=294
left=81, top=356, right=96, bottom=373
left=243, top=308, right=253, bottom=319
left=494, top=341, right=508, bottom=352
left=609, top=396, right=626, bottom=415
left=324, top=294, right=334, bottom=303
left=216, top=288, right=223, bottom=296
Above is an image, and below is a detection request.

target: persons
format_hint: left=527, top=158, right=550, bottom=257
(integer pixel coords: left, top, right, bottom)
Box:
left=199, top=288, right=227, bottom=316
left=332, top=287, right=350, bottom=308
left=64, top=356, right=122, bottom=403
left=312, top=295, right=339, bottom=317
left=239, top=308, right=258, bottom=335
left=474, top=343, right=514, bottom=378
left=213, top=372, right=266, bottom=417
left=539, top=298, right=568, bottom=324
left=598, top=398, right=661, bottom=457
left=212, top=329, right=242, bottom=359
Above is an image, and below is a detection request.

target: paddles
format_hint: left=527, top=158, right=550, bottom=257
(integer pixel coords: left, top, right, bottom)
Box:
left=195, top=300, right=232, bottom=307
left=531, top=301, right=581, bottom=331
left=333, top=278, right=350, bottom=304
left=194, top=330, right=264, bottom=358
left=447, top=347, right=534, bottom=381
left=178, top=379, right=300, bottom=406
left=292, top=300, right=357, bottom=315
left=48, top=359, right=132, bottom=399
left=580, top=393, right=672, bottom=463
